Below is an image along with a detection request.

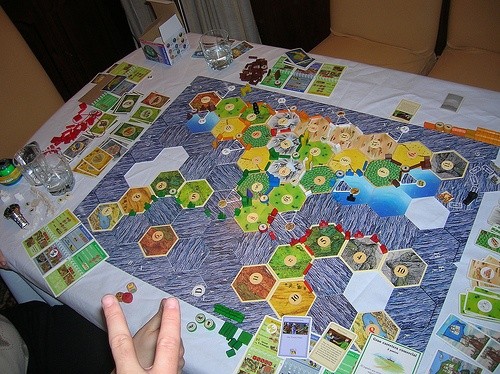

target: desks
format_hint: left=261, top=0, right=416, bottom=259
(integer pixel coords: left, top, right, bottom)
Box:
left=0, top=31, right=500, bottom=373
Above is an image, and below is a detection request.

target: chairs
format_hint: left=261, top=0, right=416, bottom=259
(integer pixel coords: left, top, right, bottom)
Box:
left=305, top=0, right=444, bottom=78
left=427, top=0, right=500, bottom=94
left=0, top=8, right=66, bottom=163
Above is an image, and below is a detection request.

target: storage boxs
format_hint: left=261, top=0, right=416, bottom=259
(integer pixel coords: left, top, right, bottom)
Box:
left=135, top=0, right=191, bottom=67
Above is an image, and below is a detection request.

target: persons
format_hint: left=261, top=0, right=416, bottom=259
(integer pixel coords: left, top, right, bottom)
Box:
left=0, top=253, right=184, bottom=374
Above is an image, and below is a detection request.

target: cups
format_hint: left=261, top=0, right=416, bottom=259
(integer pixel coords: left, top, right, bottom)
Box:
left=199, top=29, right=233, bottom=71
left=13, top=140, right=49, bottom=187
left=31, top=148, right=75, bottom=196
